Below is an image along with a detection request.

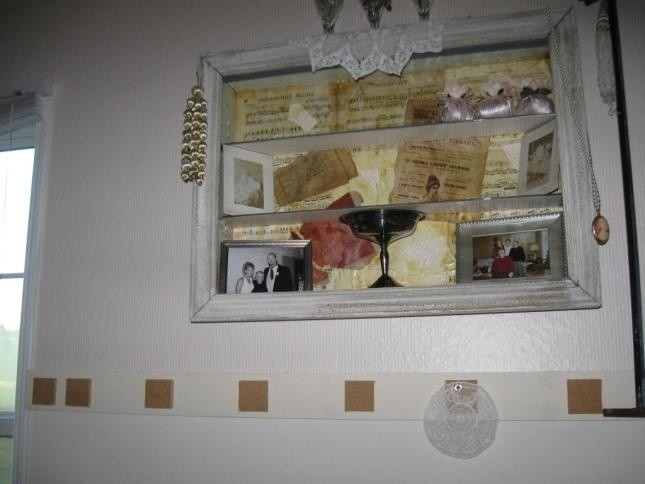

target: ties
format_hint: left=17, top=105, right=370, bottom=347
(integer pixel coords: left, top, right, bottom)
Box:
left=270, top=267, right=274, bottom=280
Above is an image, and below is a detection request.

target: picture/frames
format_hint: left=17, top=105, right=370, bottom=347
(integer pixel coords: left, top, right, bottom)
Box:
left=219, top=142, right=277, bottom=214
left=454, top=211, right=567, bottom=282
left=219, top=237, right=314, bottom=295
left=519, top=118, right=560, bottom=196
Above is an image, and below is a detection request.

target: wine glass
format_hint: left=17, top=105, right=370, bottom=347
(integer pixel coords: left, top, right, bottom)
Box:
left=340, top=208, right=426, bottom=292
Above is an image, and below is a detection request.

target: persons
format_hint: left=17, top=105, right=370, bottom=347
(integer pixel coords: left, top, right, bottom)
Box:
left=491, top=239, right=526, bottom=279
left=263, top=253, right=296, bottom=293
left=252, top=271, right=269, bottom=294
left=422, top=175, right=440, bottom=202
left=235, top=261, right=253, bottom=293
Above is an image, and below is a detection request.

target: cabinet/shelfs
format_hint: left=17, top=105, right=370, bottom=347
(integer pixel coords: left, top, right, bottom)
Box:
left=217, top=110, right=562, bottom=215
